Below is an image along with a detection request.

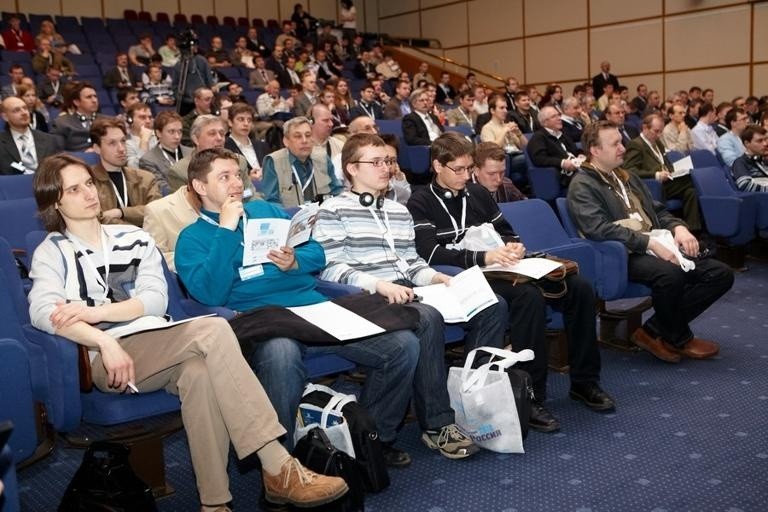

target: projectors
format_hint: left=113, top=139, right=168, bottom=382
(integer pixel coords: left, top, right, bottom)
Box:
left=310, top=19, right=335, bottom=29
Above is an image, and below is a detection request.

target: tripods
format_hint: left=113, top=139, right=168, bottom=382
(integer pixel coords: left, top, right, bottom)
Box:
left=176, top=48, right=206, bottom=114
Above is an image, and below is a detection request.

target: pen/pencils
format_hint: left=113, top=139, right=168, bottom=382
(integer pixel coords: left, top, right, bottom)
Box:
left=668, top=175, right=674, bottom=181
left=128, top=381, right=139, bottom=393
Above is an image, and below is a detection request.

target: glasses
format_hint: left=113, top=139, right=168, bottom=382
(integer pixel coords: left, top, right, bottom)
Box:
left=445, top=164, right=476, bottom=175
left=351, top=159, right=391, bottom=167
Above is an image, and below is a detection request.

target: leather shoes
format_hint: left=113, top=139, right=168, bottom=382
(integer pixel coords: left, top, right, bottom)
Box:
left=656, top=335, right=720, bottom=359
left=630, top=328, right=682, bottom=362
left=568, top=382, right=616, bottom=411
left=528, top=400, right=562, bottom=433
left=261, top=456, right=349, bottom=508
left=384, top=447, right=412, bottom=467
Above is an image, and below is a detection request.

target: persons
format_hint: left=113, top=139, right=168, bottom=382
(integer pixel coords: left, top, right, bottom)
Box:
left=568, top=120, right=734, bottom=361
left=1, top=0, right=767, bottom=280
left=173, top=147, right=420, bottom=510
left=27, top=152, right=349, bottom=508
left=312, top=136, right=510, bottom=460
left=405, top=130, right=617, bottom=430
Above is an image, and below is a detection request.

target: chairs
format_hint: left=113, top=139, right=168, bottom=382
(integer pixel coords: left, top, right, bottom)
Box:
left=0, top=10, right=346, bottom=512
left=348, top=25, right=768, bottom=361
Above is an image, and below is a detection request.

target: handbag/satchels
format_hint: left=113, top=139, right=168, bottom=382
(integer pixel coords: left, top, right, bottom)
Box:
left=58, top=438, right=160, bottom=511
left=293, top=391, right=391, bottom=512
left=447, top=356, right=534, bottom=455
left=486, top=253, right=579, bottom=284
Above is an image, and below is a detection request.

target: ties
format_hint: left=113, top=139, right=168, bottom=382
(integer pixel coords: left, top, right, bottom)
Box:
left=20, top=136, right=36, bottom=169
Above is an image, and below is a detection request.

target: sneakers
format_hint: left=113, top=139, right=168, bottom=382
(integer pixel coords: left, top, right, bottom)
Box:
left=421, top=423, right=479, bottom=459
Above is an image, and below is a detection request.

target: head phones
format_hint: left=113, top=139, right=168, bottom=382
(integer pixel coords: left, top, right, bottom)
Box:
left=678, top=244, right=710, bottom=260
left=269, top=93, right=280, bottom=98
left=350, top=185, right=384, bottom=209
left=126, top=111, right=133, bottom=124
left=213, top=96, right=220, bottom=115
left=744, top=152, right=761, bottom=161
left=432, top=175, right=470, bottom=199
left=75, top=107, right=96, bottom=121
left=307, top=104, right=320, bottom=127
